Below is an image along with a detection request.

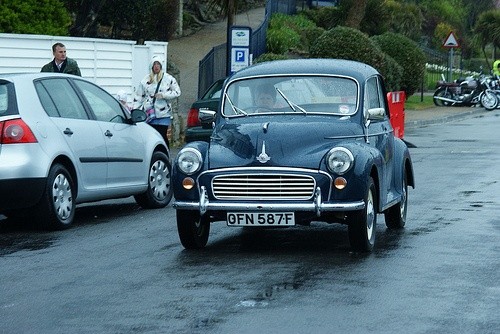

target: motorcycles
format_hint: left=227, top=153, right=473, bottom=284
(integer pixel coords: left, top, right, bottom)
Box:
left=433, top=66, right=500, bottom=111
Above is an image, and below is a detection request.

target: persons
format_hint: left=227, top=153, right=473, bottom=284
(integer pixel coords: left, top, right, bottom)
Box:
left=133, top=56, right=181, bottom=151
left=41, top=43, right=82, bottom=76
left=493, top=52, right=500, bottom=82
left=116, top=89, right=133, bottom=112
left=255, top=81, right=277, bottom=113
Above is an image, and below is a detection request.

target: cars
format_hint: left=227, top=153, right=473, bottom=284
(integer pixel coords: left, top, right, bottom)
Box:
left=0, top=71, right=173, bottom=227
left=185, top=78, right=226, bottom=145
left=171, top=59, right=416, bottom=253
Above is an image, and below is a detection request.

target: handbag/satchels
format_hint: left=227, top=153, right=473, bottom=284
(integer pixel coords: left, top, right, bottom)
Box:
left=144, top=107, right=156, bottom=123
